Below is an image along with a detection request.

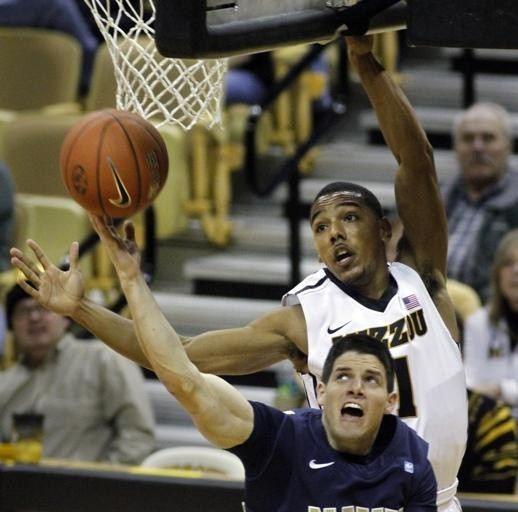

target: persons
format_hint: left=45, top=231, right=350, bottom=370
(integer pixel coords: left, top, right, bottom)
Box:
left=8, top=35, right=470, bottom=512
left=460, top=228, right=517, bottom=415
left=437, top=98, right=518, bottom=309
left=80, top=212, right=438, bottom=512
left=384, top=204, right=481, bottom=342
left=454, top=318, right=517, bottom=495
left=1, top=280, right=158, bottom=466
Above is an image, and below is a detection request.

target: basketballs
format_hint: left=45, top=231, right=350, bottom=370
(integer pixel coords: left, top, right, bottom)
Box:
left=59, top=107, right=168, bottom=219
